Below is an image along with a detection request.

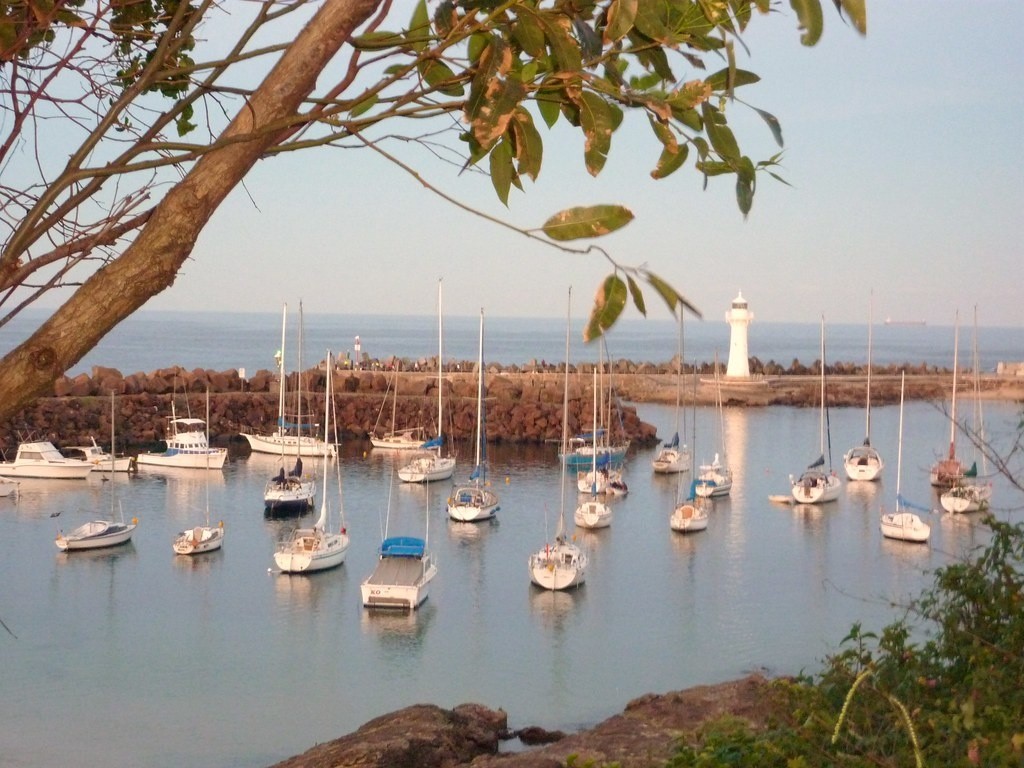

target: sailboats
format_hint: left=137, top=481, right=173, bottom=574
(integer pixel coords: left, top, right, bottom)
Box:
left=4, top=267, right=995, bottom=614
